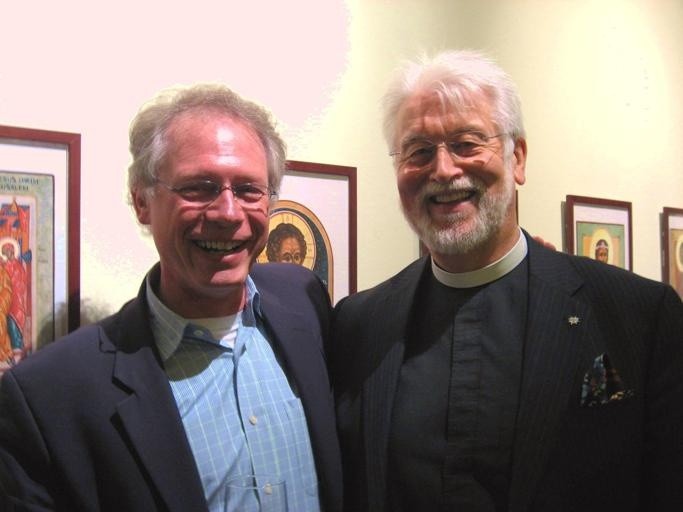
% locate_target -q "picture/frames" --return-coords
[0,124,81,381]
[563,195,683,304]
[253,160,357,318]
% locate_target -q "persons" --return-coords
[594,238,610,264]
[1,82,345,512]
[329,47,682,510]
[264,221,307,268]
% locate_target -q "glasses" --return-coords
[388,131,505,168]
[148,164,279,208]
